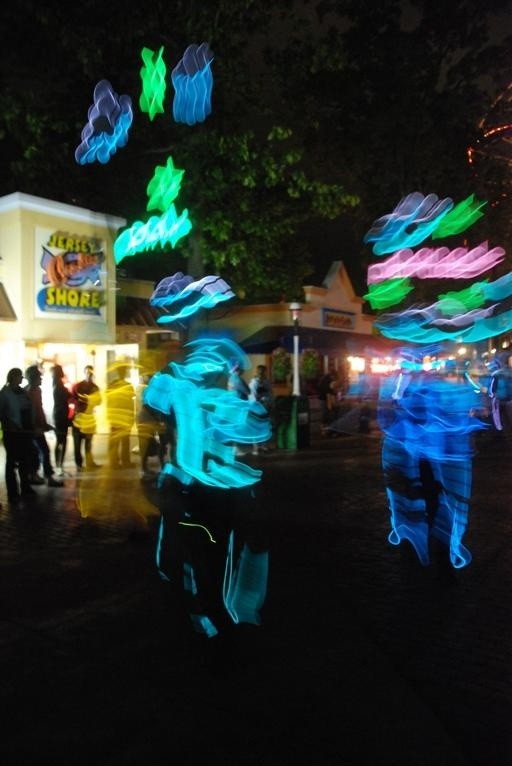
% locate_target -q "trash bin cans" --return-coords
[274,394,310,451]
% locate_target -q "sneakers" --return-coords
[48,479,64,487]
[28,477,45,484]
[20,490,35,505]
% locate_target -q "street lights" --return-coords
[287,301,304,399]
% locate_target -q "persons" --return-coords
[245,363,275,458]
[24,367,68,488]
[49,364,77,481]
[66,366,104,471]
[224,359,254,457]
[106,364,138,471]
[0,367,41,505]
[315,368,344,439]
